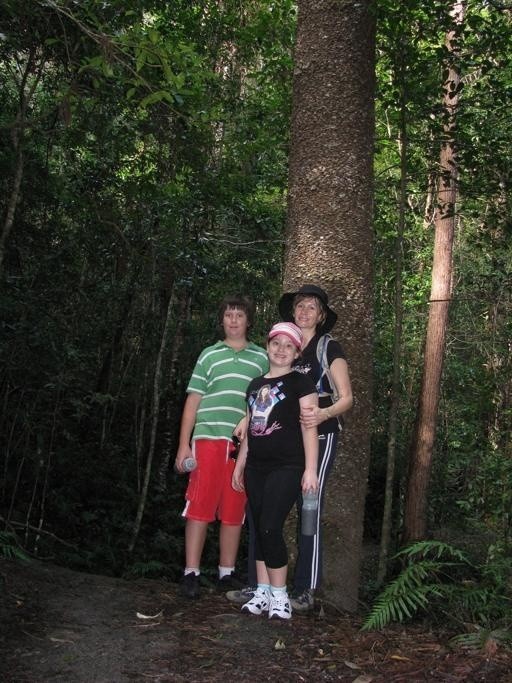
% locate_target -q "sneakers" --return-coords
[292,591,319,613]
[240,587,293,622]
[173,572,203,600]
[218,572,254,605]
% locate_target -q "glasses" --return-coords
[230,434,240,461]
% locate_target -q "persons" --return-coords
[174,295,271,600]
[232,321,320,621]
[226,284,354,615]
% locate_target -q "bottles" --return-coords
[301,485,319,536]
[173,457,197,475]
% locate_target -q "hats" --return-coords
[268,321,303,349]
[277,283,338,337]
